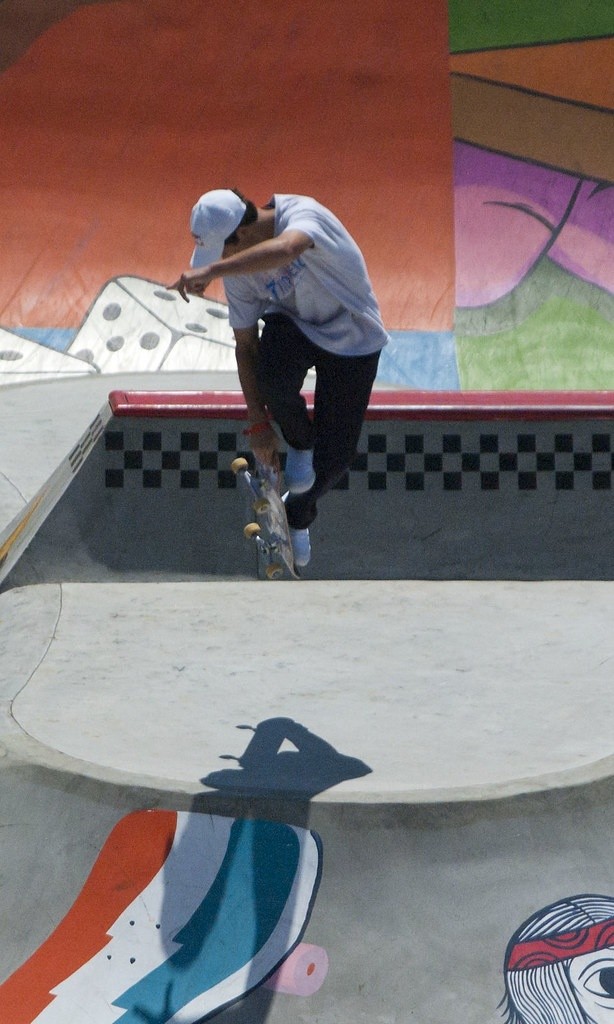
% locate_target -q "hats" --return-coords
[189,188,246,269]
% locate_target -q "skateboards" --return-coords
[228,451,303,583]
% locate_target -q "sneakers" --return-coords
[280,490,311,566]
[284,446,316,493]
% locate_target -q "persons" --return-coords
[166,189,392,568]
